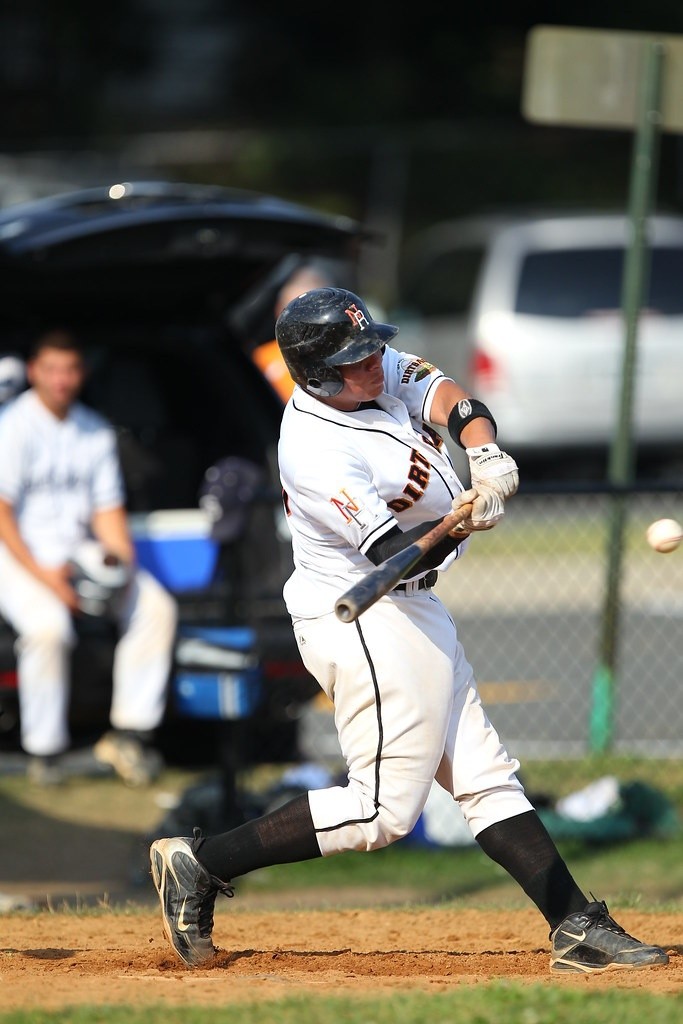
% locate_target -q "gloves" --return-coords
[465,443,520,503]
[443,486,505,535]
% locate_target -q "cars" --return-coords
[1,179,373,493]
[362,205,683,479]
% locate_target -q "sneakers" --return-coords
[548,890,670,972]
[151,826,236,971]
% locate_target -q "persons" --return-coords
[133,258,370,857]
[148,289,670,978]
[0,328,179,791]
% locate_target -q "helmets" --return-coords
[276,287,399,398]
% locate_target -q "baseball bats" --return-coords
[333,500,473,624]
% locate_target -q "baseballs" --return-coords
[646,518,683,553]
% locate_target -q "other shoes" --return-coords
[94,730,162,786]
[27,752,67,787]
